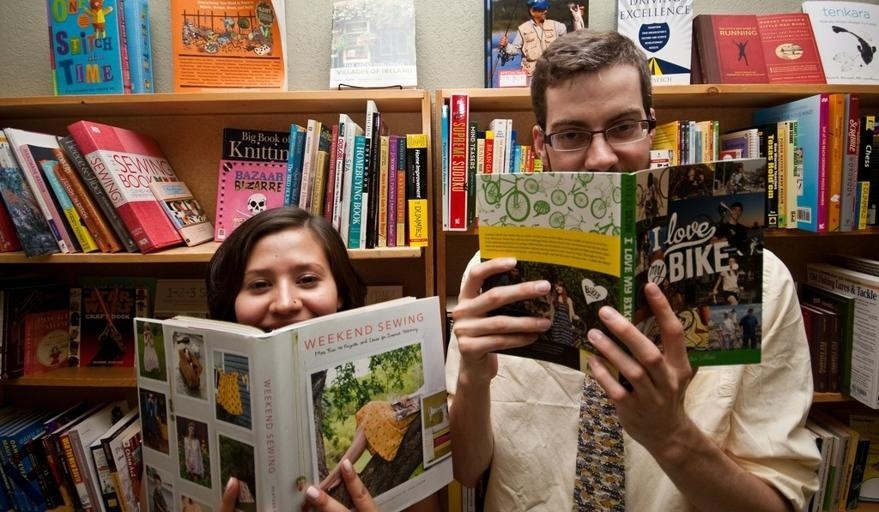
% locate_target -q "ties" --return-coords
[571,374,626,512]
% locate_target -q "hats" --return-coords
[730,201,745,209]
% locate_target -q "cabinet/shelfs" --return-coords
[436,85,875,512]
[0,89,433,512]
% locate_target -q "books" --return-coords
[67,278,155,368]
[46,1,155,96]
[282,101,429,249]
[134,296,452,512]
[800,2,879,86]
[331,3,418,89]
[214,128,292,245]
[172,2,287,93]
[0,400,143,512]
[767,94,878,233]
[442,98,476,233]
[758,15,827,85]
[0,278,69,380]
[796,257,879,410]
[806,414,879,511]
[484,1,588,87]
[694,15,767,84]
[0,122,214,258]
[479,118,544,173]
[650,122,768,160]
[480,159,765,389]
[158,279,207,321]
[618,1,693,85]
[362,285,404,304]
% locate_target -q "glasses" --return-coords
[542,115,659,153]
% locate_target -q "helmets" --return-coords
[526,0,550,11]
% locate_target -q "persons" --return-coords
[545,279,581,346]
[725,164,752,194]
[718,312,736,351]
[177,337,201,388]
[713,259,740,306]
[222,453,256,512]
[318,385,423,490]
[718,205,751,238]
[501,0,583,86]
[153,474,168,512]
[143,321,161,373]
[143,392,161,446]
[739,309,760,351]
[184,420,204,480]
